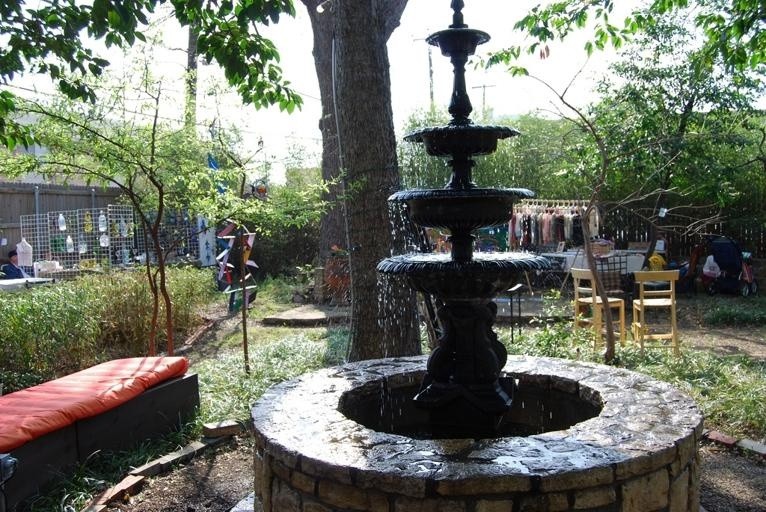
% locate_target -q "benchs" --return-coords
[0,356,199,511]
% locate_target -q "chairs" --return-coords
[569,267,681,357]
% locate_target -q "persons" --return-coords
[2,250,31,278]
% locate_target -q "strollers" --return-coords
[695,230,758,299]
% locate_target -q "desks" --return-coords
[541,249,666,290]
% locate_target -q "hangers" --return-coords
[518,199,594,214]
